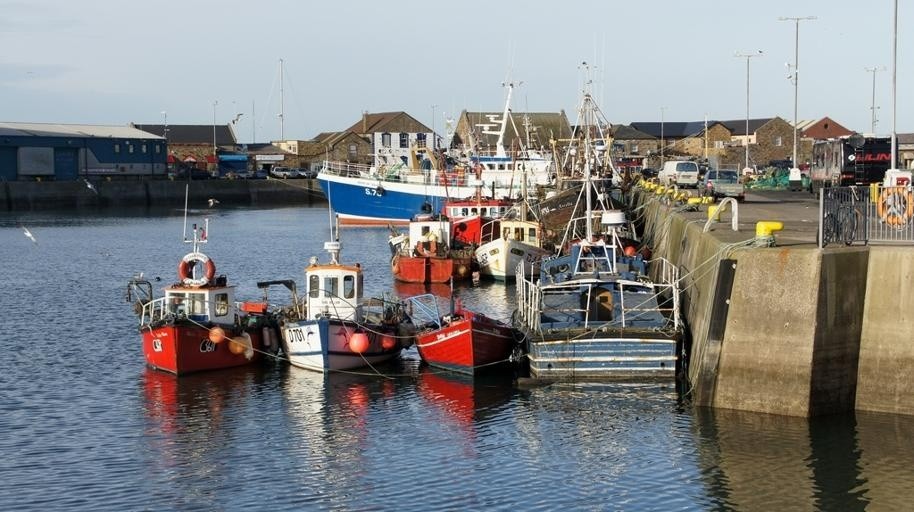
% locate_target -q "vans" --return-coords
[658,161,699,187]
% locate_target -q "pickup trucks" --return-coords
[697,168,746,204]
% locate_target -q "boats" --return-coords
[125,187,275,377]
[314,81,560,227]
[277,142,399,374]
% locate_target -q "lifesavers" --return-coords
[877,186,914,225]
[178,252,215,288]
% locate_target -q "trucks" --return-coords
[809,136,899,196]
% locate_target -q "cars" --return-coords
[179,165,209,179]
[256,170,267,179]
[272,164,311,180]
[641,168,658,179]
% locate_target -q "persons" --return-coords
[474,164,482,180]
[581,246,594,270]
[417,241,438,257]
[515,231,520,240]
[504,228,509,240]
[386,303,400,323]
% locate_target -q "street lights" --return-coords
[736,50,766,167]
[779,15,817,167]
[864,64,883,135]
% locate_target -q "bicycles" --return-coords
[816,192,859,247]
